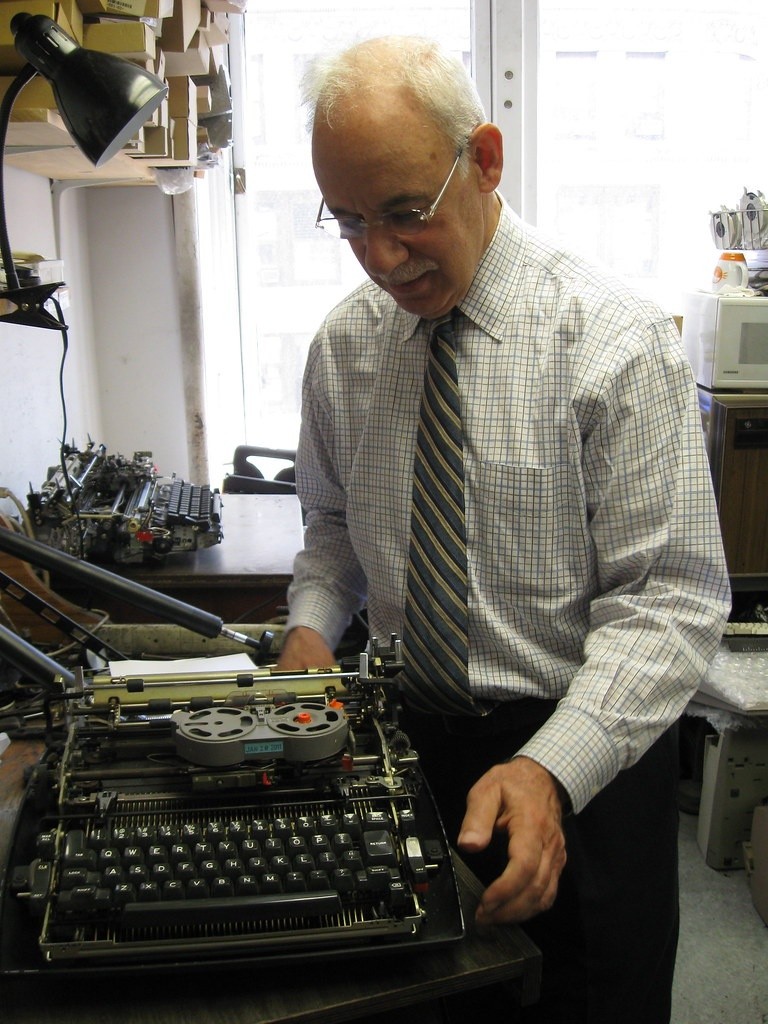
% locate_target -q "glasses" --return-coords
[315,150,462,238]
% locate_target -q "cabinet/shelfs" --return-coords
[38,493,304,625]
[696,388,768,594]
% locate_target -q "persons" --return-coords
[277,38,731,1024]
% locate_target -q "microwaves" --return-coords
[681,291,768,392]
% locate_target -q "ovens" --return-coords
[696,386,768,592]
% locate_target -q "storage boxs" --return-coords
[0,0,245,168]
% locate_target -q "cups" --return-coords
[713,253,748,295]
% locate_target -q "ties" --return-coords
[399,316,482,721]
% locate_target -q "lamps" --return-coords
[0,11,169,330]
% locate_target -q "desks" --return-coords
[0,732,543,1023]
